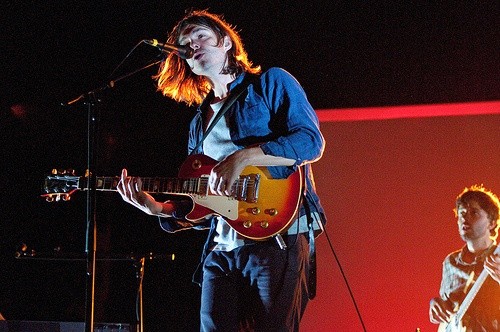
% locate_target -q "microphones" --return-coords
[143,39,195,59]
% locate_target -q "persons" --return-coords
[115,9,326,332]
[427,185,500,332]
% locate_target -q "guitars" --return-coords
[436,240,500,332]
[43,151,306,241]
